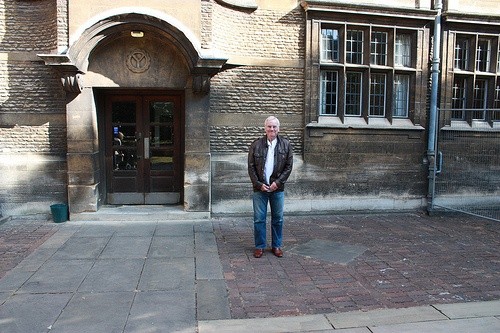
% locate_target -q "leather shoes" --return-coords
[272,248,283,257]
[254,248,263,257]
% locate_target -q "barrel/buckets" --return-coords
[50,204,68,223]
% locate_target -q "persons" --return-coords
[112,126,160,172]
[247,115,293,258]
